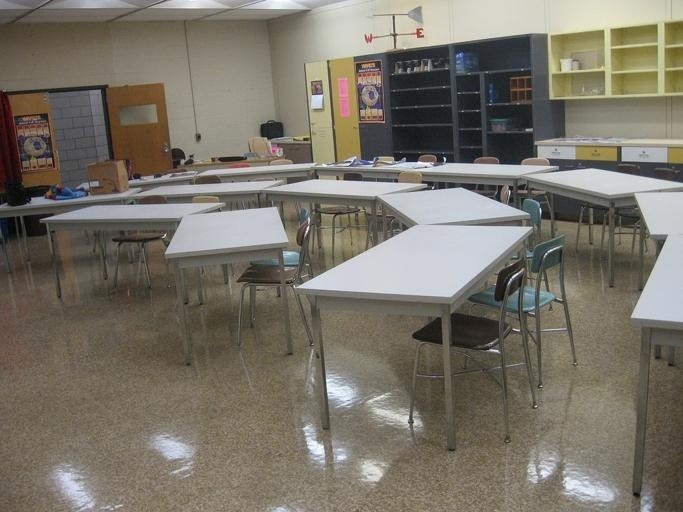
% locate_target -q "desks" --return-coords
[271,140,313,164]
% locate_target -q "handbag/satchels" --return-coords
[259,119,285,140]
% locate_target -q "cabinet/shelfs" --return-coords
[547,19,682,101]
[451,33,565,165]
[384,42,454,161]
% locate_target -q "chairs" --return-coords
[417,155,438,163]
[374,157,394,162]
[172,148,185,168]
